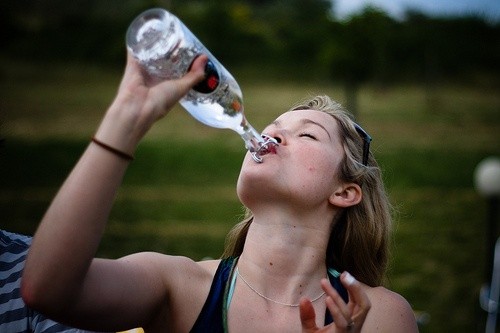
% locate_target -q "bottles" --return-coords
[124,9,279,162]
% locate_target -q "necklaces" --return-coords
[236,268,326,307]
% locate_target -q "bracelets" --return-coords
[89,135,134,160]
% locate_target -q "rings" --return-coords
[348,320,353,331]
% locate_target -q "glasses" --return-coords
[351,121,372,182]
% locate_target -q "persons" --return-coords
[0,230,93,333]
[20,47,420,333]
